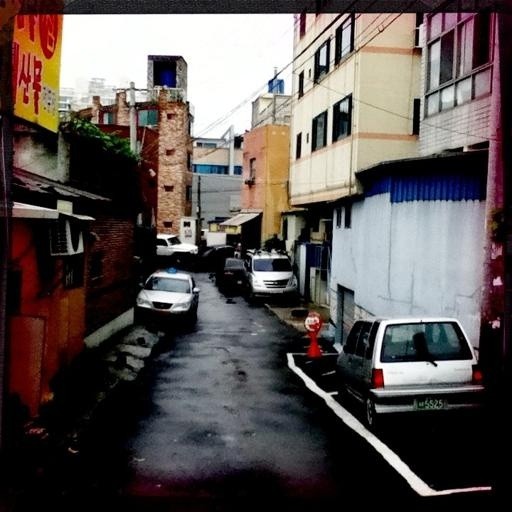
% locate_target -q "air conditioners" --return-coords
[49,219,85,256]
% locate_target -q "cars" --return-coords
[157,234,200,269]
[135,267,200,329]
[336,317,487,432]
[200,234,299,301]
